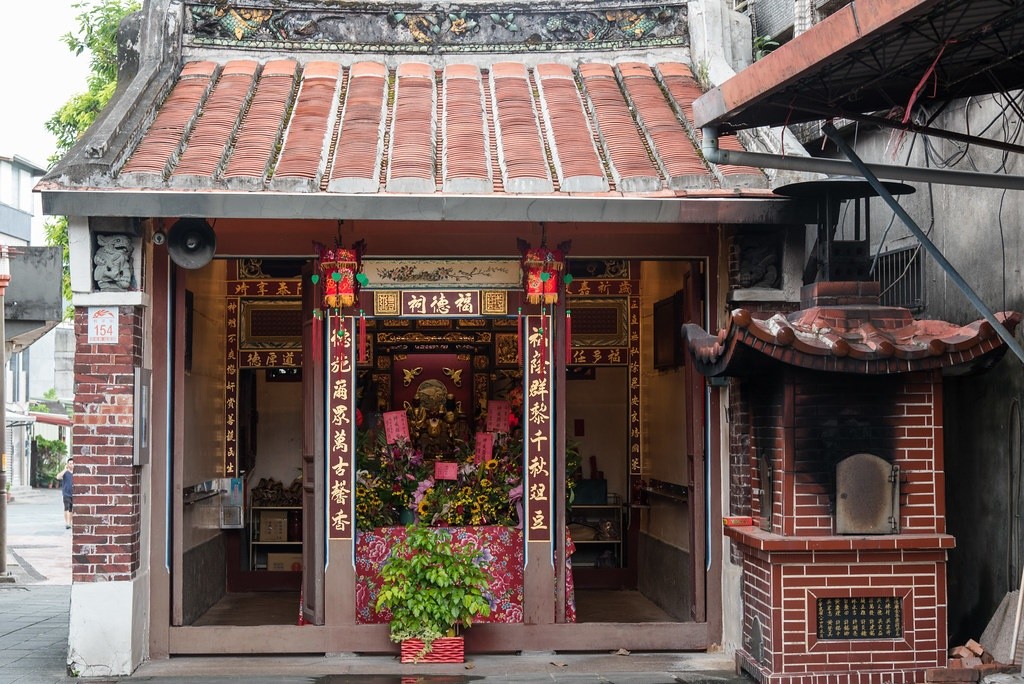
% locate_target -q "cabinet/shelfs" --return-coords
[250,494,303,572]
[565,504,623,568]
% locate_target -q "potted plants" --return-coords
[373,523,496,662]
[34,468,59,488]
[6,481,12,502]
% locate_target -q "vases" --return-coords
[399,509,415,526]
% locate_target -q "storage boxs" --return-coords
[257,510,288,542]
[268,553,303,572]
[723,516,752,526]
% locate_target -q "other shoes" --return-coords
[65,523,70,529]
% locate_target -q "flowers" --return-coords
[355,385,524,532]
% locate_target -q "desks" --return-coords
[298,525,575,624]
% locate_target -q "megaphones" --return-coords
[166,217,217,269]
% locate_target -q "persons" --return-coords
[56,458,74,529]
